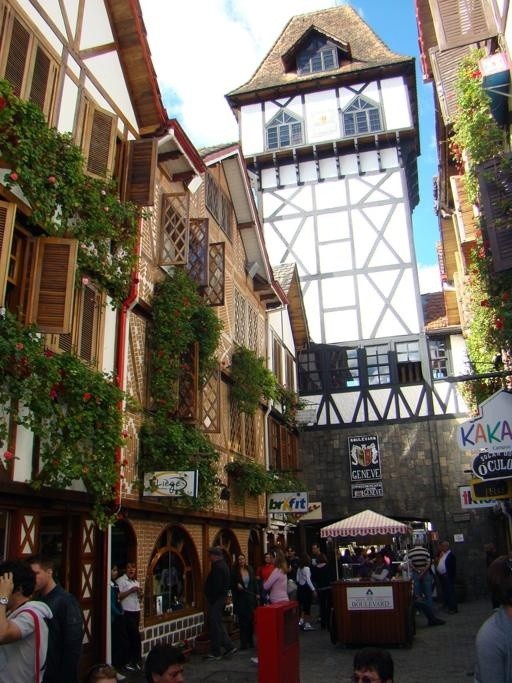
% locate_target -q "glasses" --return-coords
[350,674,390,683]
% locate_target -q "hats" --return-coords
[207,547,224,556]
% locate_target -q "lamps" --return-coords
[210,473,230,501]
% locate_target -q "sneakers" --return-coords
[427,616,447,627]
[222,647,239,660]
[202,653,222,663]
[297,618,321,632]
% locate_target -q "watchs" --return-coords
[1,595,9,606]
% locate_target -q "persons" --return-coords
[0,557,53,683]
[116,561,145,671]
[472,555,511,683]
[111,563,130,681]
[351,646,393,683]
[26,553,83,682]
[146,643,186,683]
[201,539,460,660]
[84,663,117,683]
